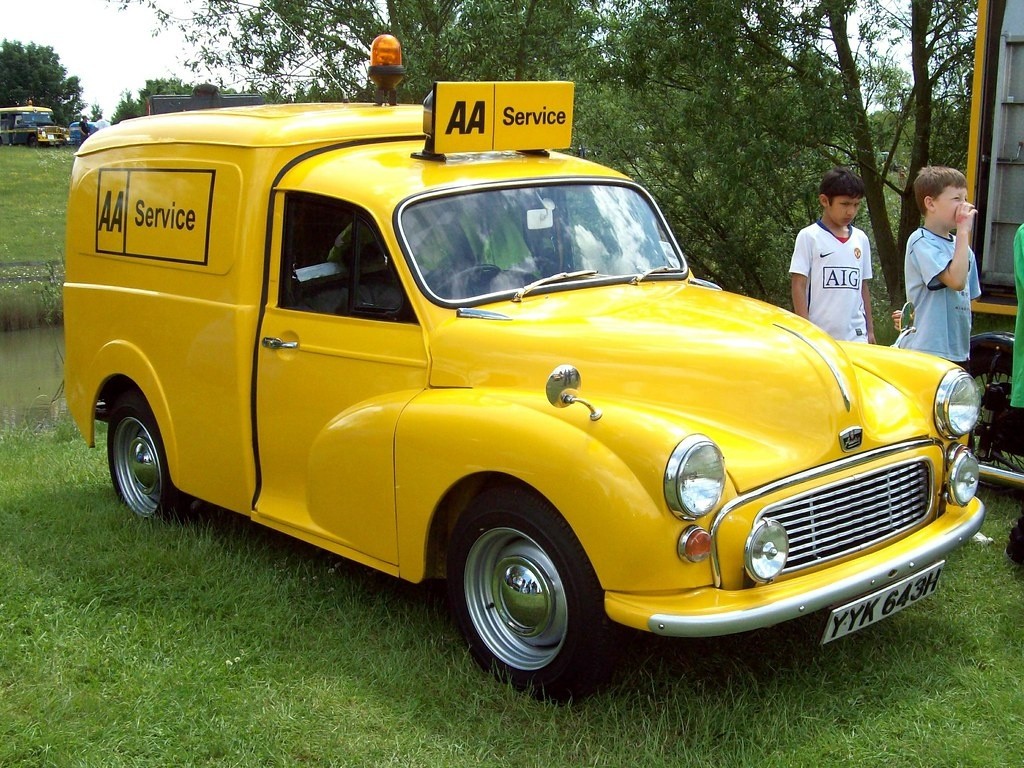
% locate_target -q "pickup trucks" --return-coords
[57,28,989,686]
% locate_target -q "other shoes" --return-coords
[971,531,990,545]
[1002,536,1023,562]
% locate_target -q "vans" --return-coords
[0,100,67,149]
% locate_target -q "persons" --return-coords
[78,116,89,142]
[93,114,107,132]
[1000,222,1024,568]
[787,166,878,345]
[890,165,995,550]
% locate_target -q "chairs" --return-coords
[327,217,422,316]
